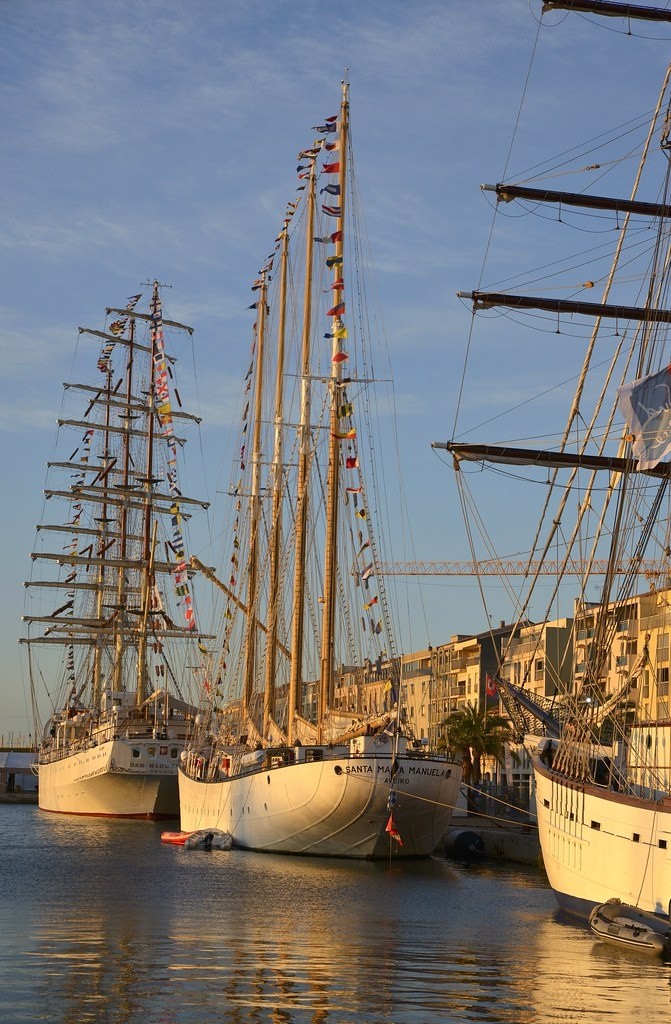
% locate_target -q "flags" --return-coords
[617,363,671,471]
[67,166,405,849]
[486,674,498,696]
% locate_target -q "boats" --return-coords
[588,897,671,956]
[161,828,232,851]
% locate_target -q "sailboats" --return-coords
[424,0,671,928]
[166,64,464,859]
[11,280,221,821]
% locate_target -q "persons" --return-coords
[50,726,56,737]
